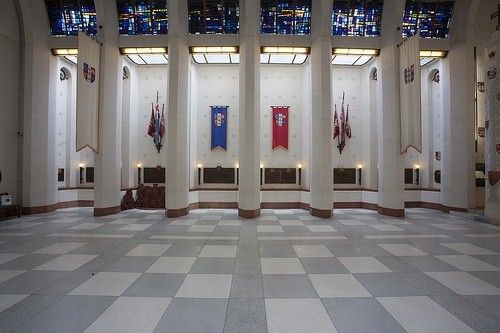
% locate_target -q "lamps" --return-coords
[358,165,362,185]
[79,163,84,184]
[137,164,142,184]
[298,164,302,185]
[198,164,202,185]
[260,165,263,185]
[416,165,420,185]
[236,163,239,185]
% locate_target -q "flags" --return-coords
[211,106,227,152]
[345,107,351,140]
[76,29,100,154]
[337,101,345,154]
[148,107,154,137]
[272,107,288,151]
[159,108,165,138]
[333,111,340,139]
[153,97,160,150]
[398,30,422,154]
